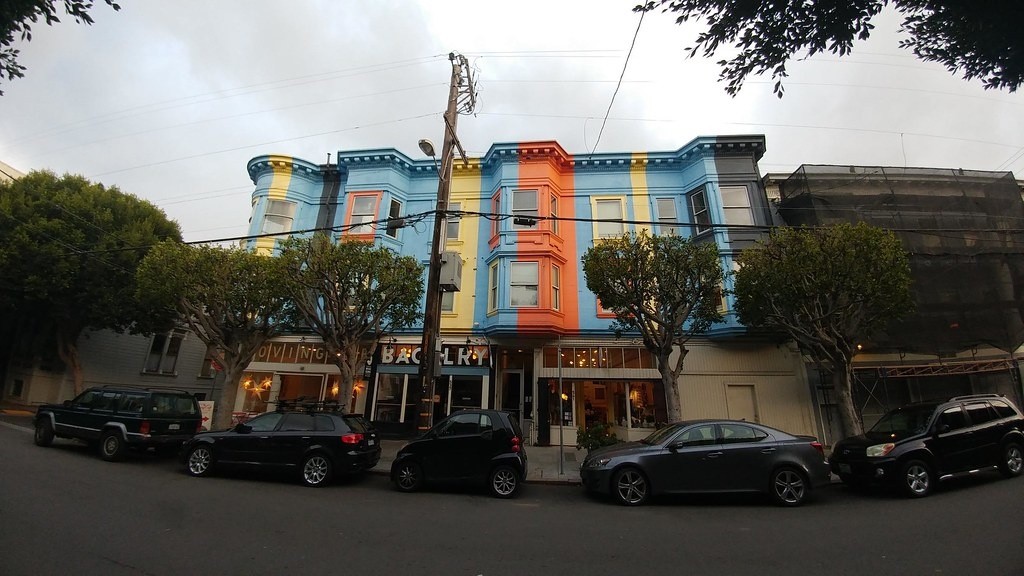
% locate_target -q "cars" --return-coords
[391,409,527,498]
[582,419,831,505]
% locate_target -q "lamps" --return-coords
[463,336,471,355]
[386,336,397,350]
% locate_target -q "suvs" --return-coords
[32,384,203,461]
[179,397,382,486]
[828,394,1024,497]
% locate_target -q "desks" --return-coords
[231,412,246,427]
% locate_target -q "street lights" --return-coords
[420,137,448,435]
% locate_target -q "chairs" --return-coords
[247,411,259,419]
[686,429,703,440]
